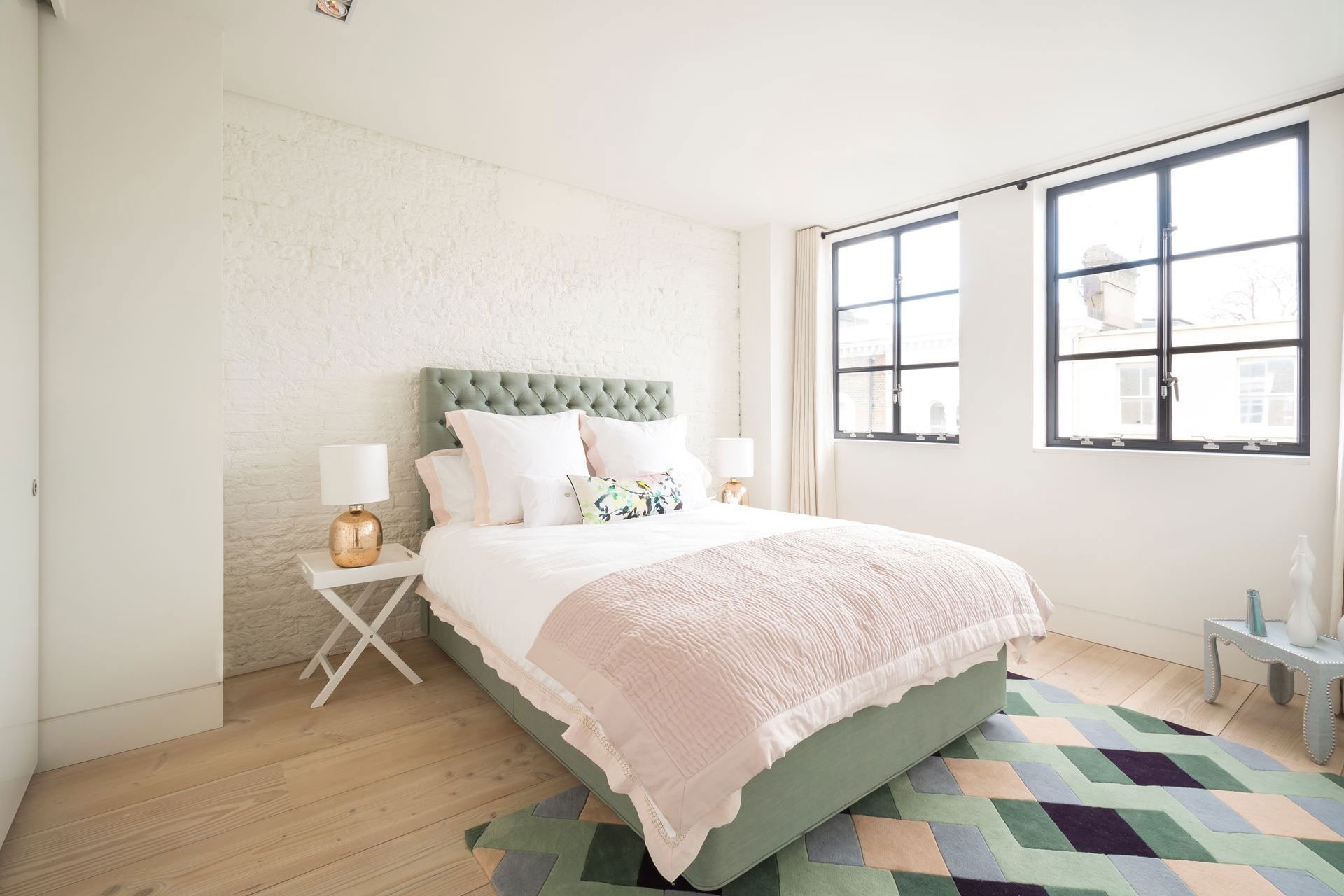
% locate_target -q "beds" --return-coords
[415,367,1055,891]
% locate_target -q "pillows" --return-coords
[414,409,713,529]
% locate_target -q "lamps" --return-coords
[716,438,754,506]
[318,444,390,568]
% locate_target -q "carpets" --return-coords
[465,669,1344,896]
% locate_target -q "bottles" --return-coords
[1246,588,1266,637]
[1291,534,1323,641]
[1286,552,1318,649]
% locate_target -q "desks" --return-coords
[297,543,426,709]
[1203,618,1344,765]
